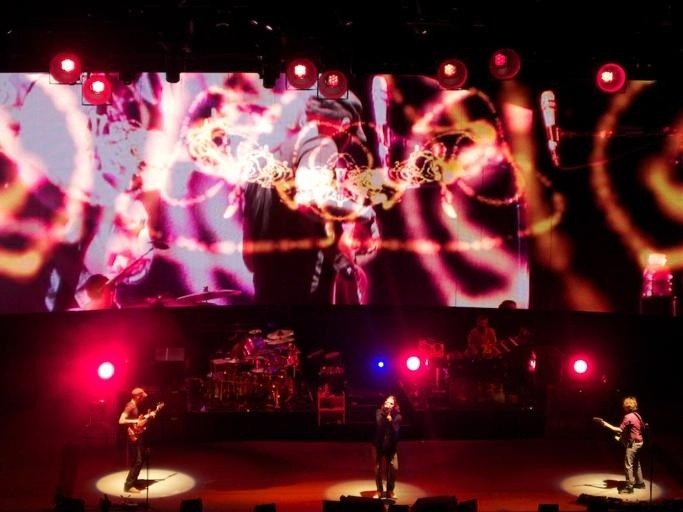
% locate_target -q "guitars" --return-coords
[127,402,165,443]
[592,417,629,447]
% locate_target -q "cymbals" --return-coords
[266,329,294,339]
[264,338,295,345]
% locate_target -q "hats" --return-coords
[131,388,148,398]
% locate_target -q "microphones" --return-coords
[372,76,391,167]
[537,90,561,168]
[151,241,169,249]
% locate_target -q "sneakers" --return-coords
[124,486,140,494]
[373,488,398,500]
[622,483,647,492]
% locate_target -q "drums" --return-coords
[241,355,265,375]
[211,358,241,385]
[269,352,289,378]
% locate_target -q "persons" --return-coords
[466,314,496,403]
[369,395,402,499]
[118,388,158,492]
[594,396,649,494]
